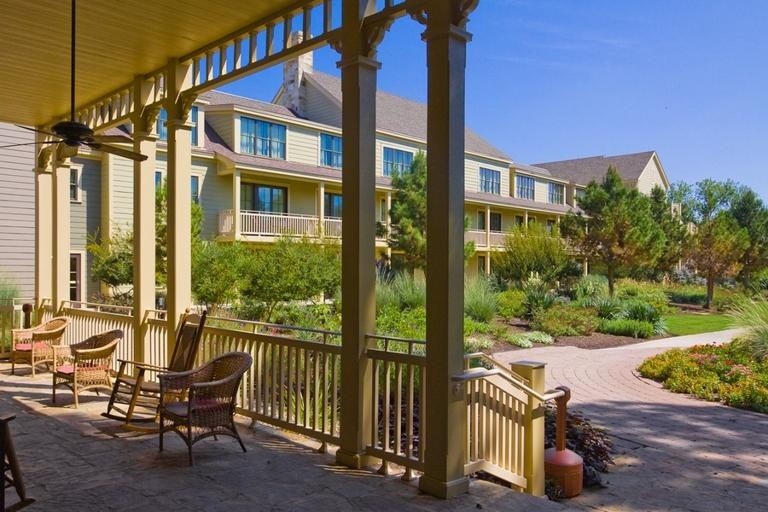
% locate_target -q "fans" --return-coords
[1,0,149,160]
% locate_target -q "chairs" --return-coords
[101,308,207,432]
[51,329,123,408]
[10,315,71,377]
[0,412,36,512]
[156,351,252,467]
